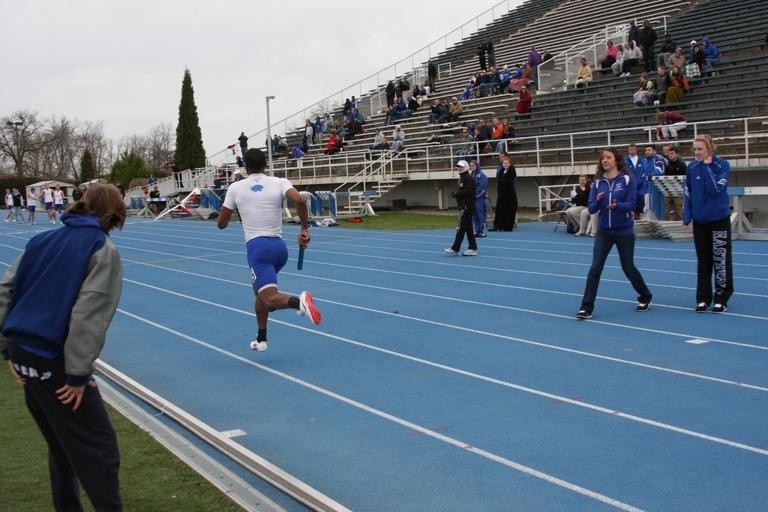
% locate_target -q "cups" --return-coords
[654,101,659,105]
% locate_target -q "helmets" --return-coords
[456,160,469,173]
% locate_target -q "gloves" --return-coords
[448,189,457,198]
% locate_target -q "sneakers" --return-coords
[712,304,727,313]
[637,296,652,313]
[575,233,585,237]
[462,250,477,256]
[576,310,592,319]
[589,233,596,236]
[696,304,708,312]
[297,292,321,325]
[250,340,266,351]
[442,248,458,256]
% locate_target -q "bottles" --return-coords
[656,135,660,140]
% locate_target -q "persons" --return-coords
[1,182,126,512]
[574,146,654,319]
[238,96,366,167]
[217,148,322,351]
[680,133,734,314]
[623,143,687,219]
[600,21,720,143]
[366,39,554,160]
[565,176,601,237]
[443,150,518,257]
[163,160,185,188]
[0,180,162,227]
[575,58,593,94]
